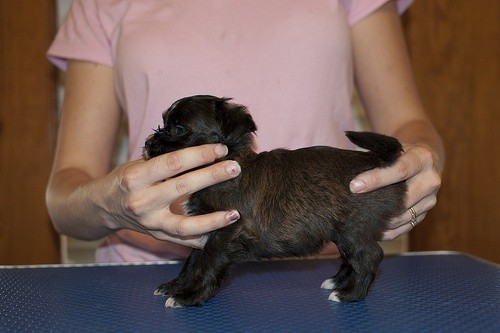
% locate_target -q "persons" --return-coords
[46,0,446,264]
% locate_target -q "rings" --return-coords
[409,207,416,221]
[411,219,419,227]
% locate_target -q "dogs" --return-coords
[144,94,408,309]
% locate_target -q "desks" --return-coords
[0,249,500,333]
